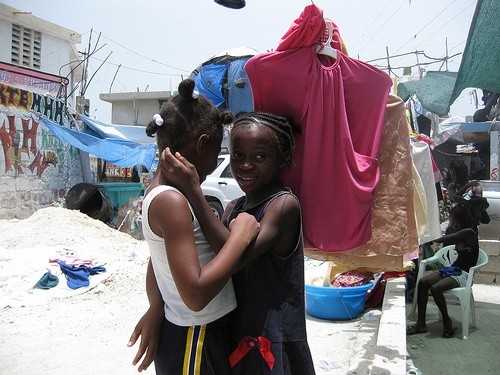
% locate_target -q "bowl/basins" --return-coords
[304,281,374,320]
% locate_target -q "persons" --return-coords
[406,204,478,339]
[441,163,483,245]
[129,77,315,375]
[439,167,451,189]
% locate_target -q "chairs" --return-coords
[408,246,488,340]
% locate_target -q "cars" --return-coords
[200,154,246,221]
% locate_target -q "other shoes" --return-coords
[407,325,427,333]
[442,329,456,338]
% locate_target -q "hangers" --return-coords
[316,17,337,59]
